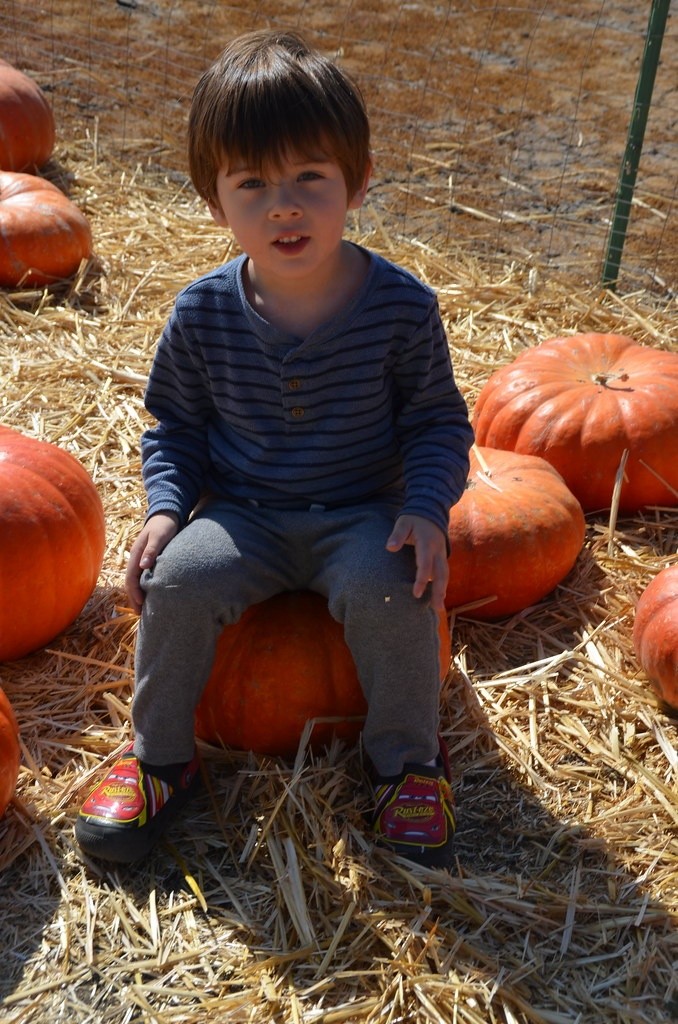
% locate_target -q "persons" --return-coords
[77,27,477,866]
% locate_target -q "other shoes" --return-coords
[357,722,458,868]
[75,740,207,863]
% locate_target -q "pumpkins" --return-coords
[0,685,21,824]
[0,57,56,175]
[0,169,93,289]
[444,447,586,620]
[633,564,678,712]
[469,332,678,513]
[192,589,452,753]
[0,424,107,662]
